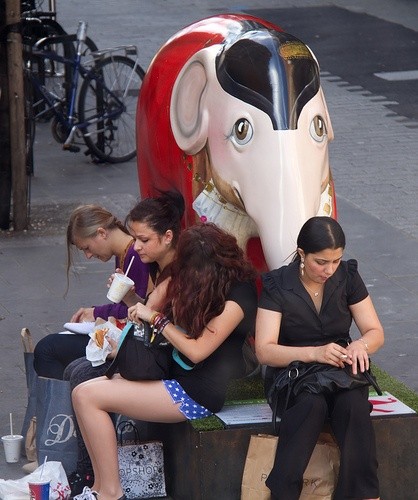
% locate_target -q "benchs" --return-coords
[148,361,418,500]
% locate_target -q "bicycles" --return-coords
[0,0,147,175]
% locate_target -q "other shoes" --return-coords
[71,485,127,500]
[21,461,38,473]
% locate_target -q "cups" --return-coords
[106,273,135,304]
[1,434,24,462]
[27,479,50,500]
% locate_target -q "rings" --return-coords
[339,354,347,360]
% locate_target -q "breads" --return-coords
[94,327,110,347]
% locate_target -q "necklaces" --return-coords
[301,279,323,296]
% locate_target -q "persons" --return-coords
[70,223,258,500]
[63,189,185,496]
[255,216,384,500]
[21,204,152,473]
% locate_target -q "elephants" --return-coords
[134,12,340,349]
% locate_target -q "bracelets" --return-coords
[359,338,368,351]
[150,313,170,334]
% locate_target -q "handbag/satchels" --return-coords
[269,337,382,436]
[0,327,167,500]
[104,322,195,382]
